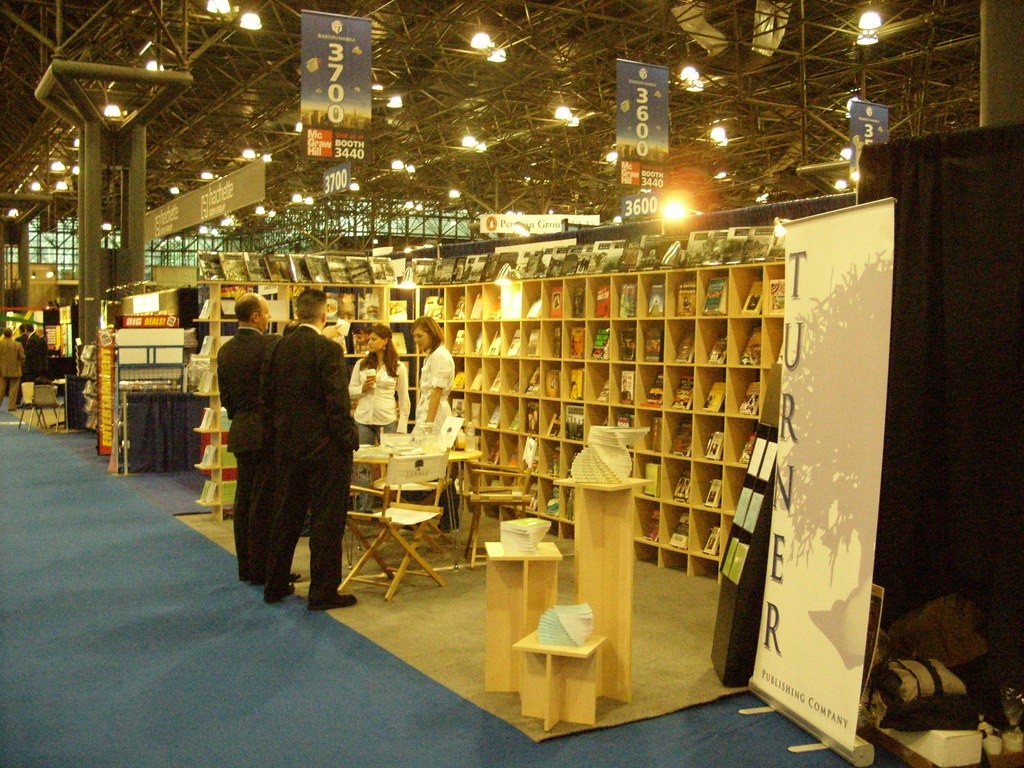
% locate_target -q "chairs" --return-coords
[27,385,66,433]
[458,436,536,570]
[19,382,48,430]
[338,426,451,601]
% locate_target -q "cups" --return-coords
[335,318,350,336]
[365,368,377,388]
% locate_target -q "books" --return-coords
[197,298,216,507]
[219,235,783,556]
[198,250,226,281]
[79,345,98,432]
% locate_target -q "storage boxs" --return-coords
[875,722,984,767]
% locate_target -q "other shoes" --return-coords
[291,573,301,581]
[307,592,357,611]
[264,583,295,603]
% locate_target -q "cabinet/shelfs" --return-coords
[80,262,786,588]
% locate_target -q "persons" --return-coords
[282,319,301,335]
[348,324,412,514]
[263,290,357,613]
[0,323,42,413]
[411,316,459,532]
[217,293,303,584]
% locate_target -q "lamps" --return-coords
[31,271,37,279]
[661,240,685,268]
[513,222,531,237]
[401,267,417,289]
[494,263,516,286]
[773,218,788,238]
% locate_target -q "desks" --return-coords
[346,435,482,569]
[553,478,653,702]
[484,542,563,695]
[512,631,609,734]
[52,380,68,431]
